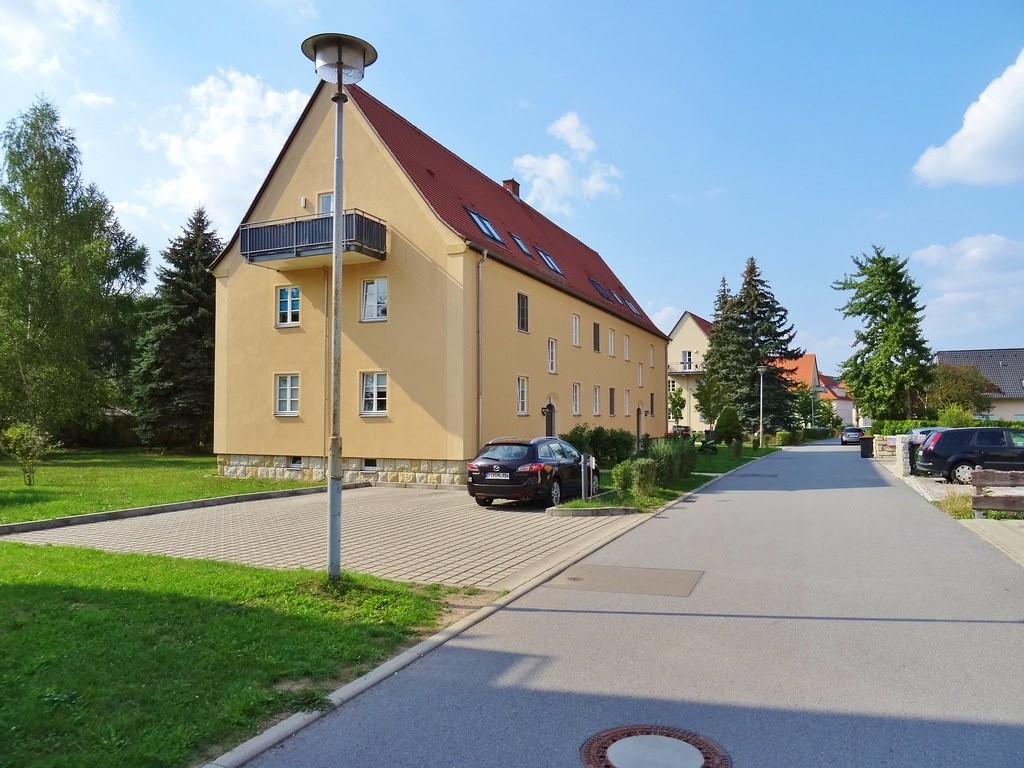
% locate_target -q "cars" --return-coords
[669,425,696,441]
[861,425,872,434]
[840,427,865,445]
[904,426,947,445]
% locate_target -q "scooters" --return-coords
[690,434,718,454]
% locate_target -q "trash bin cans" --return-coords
[858,435,875,457]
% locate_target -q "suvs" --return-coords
[464,434,601,508]
[915,424,1024,485]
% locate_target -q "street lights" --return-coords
[755,365,768,448]
[808,395,814,428]
[299,31,380,583]
